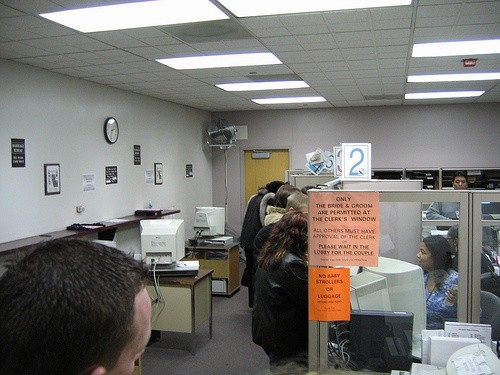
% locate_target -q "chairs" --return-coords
[481,271,500,296]
[480,289,500,340]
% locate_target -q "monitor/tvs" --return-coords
[350,256,426,341]
[140,219,185,265]
[193,207,225,236]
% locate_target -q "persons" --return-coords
[416,235,482,331]
[446,224,495,273]
[0,239,153,375]
[242,181,316,375]
[426,172,472,220]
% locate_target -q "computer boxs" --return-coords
[196,236,233,246]
[348,310,414,371]
[146,260,200,277]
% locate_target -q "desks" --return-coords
[138,269,215,355]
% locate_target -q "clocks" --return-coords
[104,116,120,144]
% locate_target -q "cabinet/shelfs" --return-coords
[182,241,241,298]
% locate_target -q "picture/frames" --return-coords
[154,162,164,185]
[43,162,62,196]
